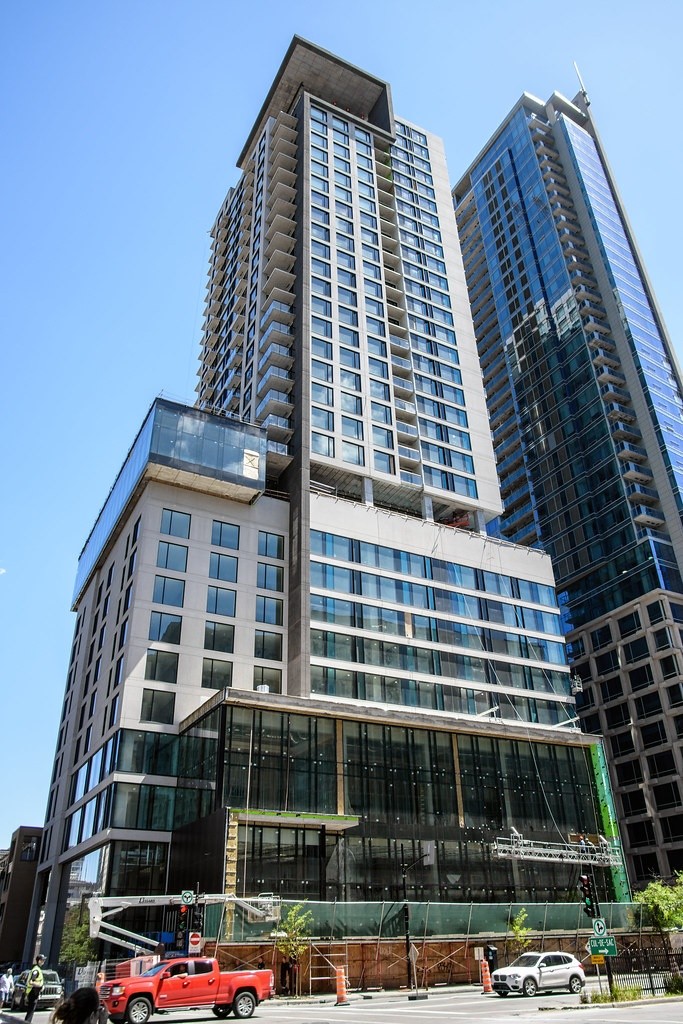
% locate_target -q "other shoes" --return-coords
[288,990,290,996]
[280,993,284,997]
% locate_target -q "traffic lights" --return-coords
[175,903,189,951]
[578,873,598,918]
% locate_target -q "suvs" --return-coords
[490,951,586,998]
[11,969,66,1011]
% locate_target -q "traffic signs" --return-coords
[589,936,618,956]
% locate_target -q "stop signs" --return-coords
[190,933,201,945]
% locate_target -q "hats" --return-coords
[36,954,47,960]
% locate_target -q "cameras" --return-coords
[97,1006,105,1020]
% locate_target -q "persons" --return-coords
[171,965,188,979]
[577,836,596,860]
[235,959,243,971]
[53,987,109,1024]
[0,968,15,1009]
[258,956,265,970]
[24,954,47,1024]
[280,956,300,997]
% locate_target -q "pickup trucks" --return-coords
[98,955,277,1024]
